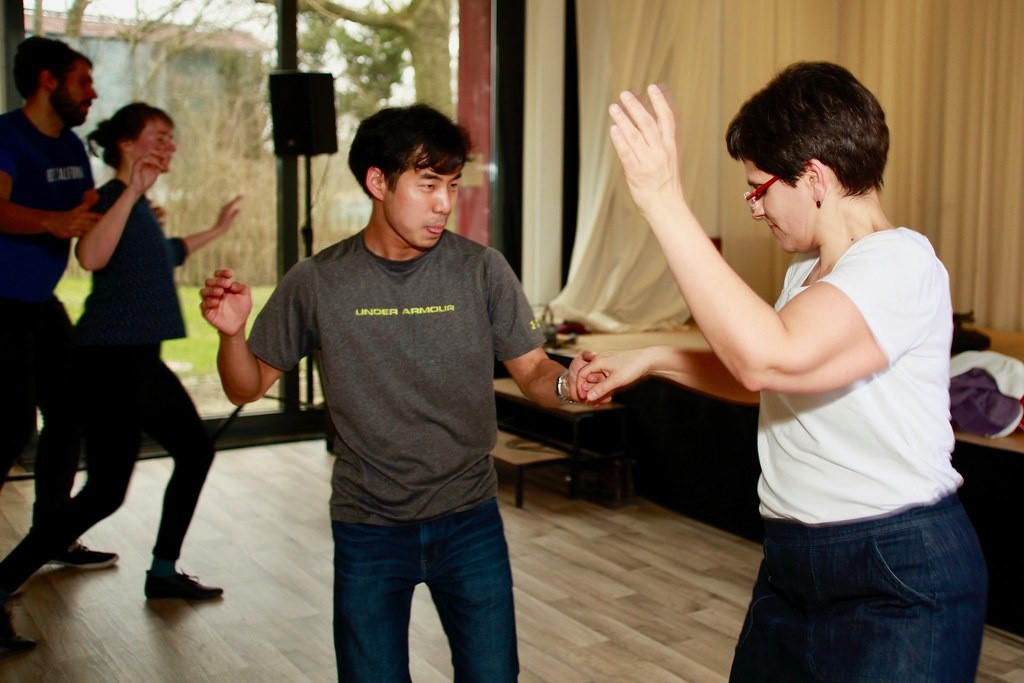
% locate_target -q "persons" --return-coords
[199,102,613,683]
[575,60,988,682]
[0,35,120,569]
[0,102,244,657]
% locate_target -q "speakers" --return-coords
[268,70,339,158]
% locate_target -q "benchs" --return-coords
[489,429,573,509]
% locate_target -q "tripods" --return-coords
[212,156,317,443]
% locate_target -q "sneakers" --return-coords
[46,538,119,568]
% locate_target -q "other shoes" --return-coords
[145,568,223,599]
[0,609,37,650]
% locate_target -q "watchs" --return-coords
[555,370,574,405]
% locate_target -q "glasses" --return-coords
[743,176,779,210]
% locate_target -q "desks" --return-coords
[493,379,624,500]
